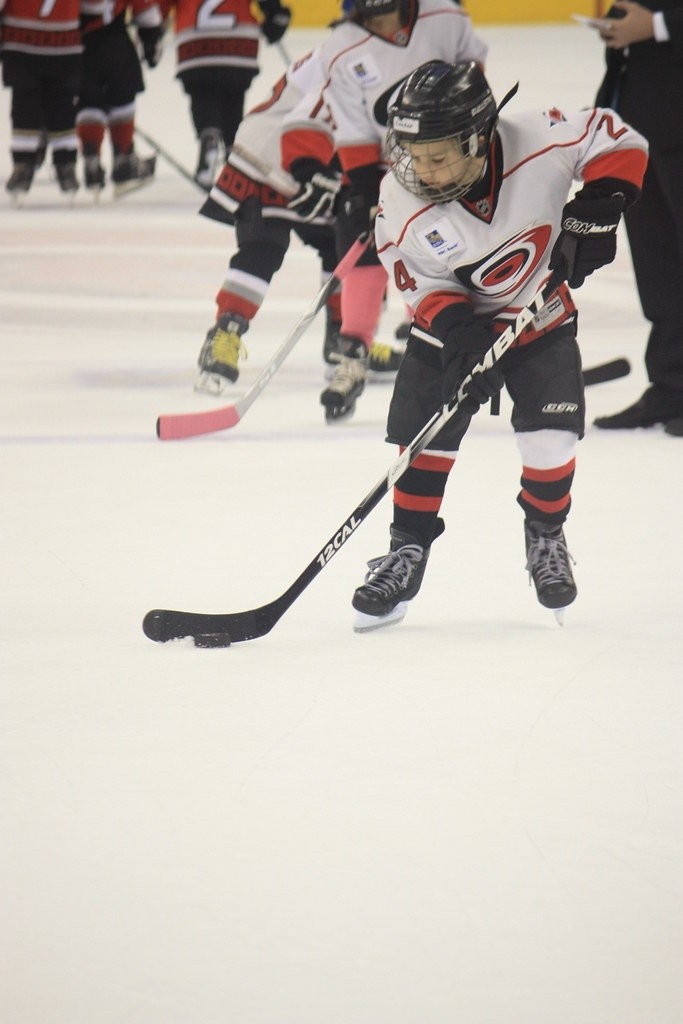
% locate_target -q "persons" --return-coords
[593,1,683,437]
[0,0,170,209]
[351,57,649,635]
[176,0,291,185]
[279,0,490,425]
[191,0,403,397]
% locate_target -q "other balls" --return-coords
[193,632,232,647]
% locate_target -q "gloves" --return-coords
[261,7,291,44]
[441,346,504,415]
[332,191,377,227]
[286,183,334,221]
[138,26,162,59]
[548,193,623,289]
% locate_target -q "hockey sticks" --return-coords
[140,268,566,643]
[132,125,209,195]
[155,225,376,439]
[275,39,294,69]
[581,358,631,385]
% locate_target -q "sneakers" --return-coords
[6,164,36,204]
[352,517,446,634]
[198,313,249,395]
[524,518,578,627]
[323,321,403,382]
[83,143,106,201]
[56,163,81,202]
[320,334,368,424]
[195,132,219,190]
[111,146,158,197]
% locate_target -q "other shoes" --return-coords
[594,382,683,429]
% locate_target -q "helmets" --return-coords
[385,60,498,205]
